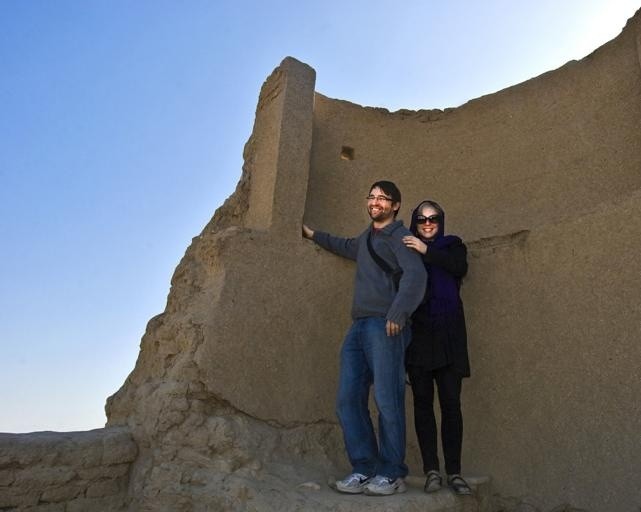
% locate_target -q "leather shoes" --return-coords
[423,472,444,494]
[447,472,472,497]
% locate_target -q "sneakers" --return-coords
[335,468,374,494]
[364,474,408,496]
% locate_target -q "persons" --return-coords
[403,200,474,495]
[303,179,429,497]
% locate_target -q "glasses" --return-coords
[414,212,442,226]
[366,191,397,204]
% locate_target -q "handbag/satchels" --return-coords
[392,271,429,307]
